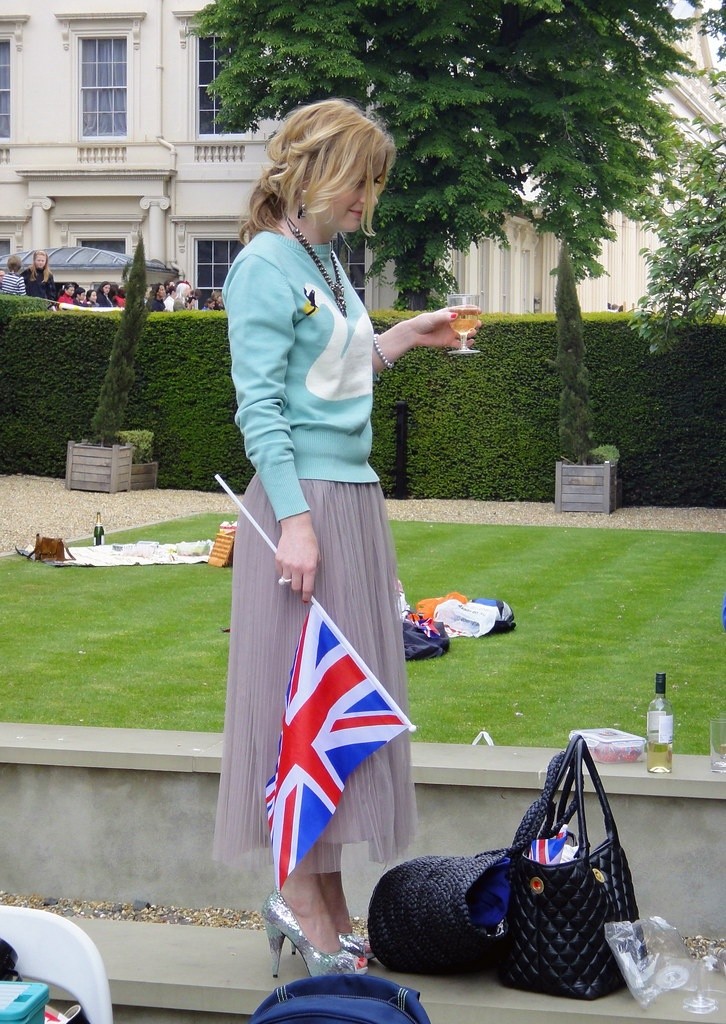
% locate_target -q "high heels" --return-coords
[261,891,377,975]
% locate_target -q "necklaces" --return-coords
[284,213,348,318]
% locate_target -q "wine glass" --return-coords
[447,294,481,354]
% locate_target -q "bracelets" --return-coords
[373,333,395,369]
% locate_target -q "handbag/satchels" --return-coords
[401,592,512,661]
[498,730,646,999]
[367,746,584,973]
[40,538,74,560]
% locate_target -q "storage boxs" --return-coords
[0,980,50,1024]
[569,728,647,763]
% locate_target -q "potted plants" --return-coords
[64,229,148,493]
[554,236,620,515]
[116,430,158,490]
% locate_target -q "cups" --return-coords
[710,719,726,773]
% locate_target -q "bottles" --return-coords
[645,673,672,774]
[93,512,104,546]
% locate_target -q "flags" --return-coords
[264,599,407,895]
[527,834,567,864]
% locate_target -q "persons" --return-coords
[58,282,75,304]
[20,250,55,301]
[97,281,116,307]
[74,287,88,307]
[208,99,480,979]
[2,256,27,296]
[0,269,6,291]
[83,290,100,307]
[111,279,225,312]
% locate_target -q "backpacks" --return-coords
[247,977,429,1024]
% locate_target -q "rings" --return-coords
[277,576,291,587]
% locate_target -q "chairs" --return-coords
[0,904,114,1024]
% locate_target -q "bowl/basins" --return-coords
[569,727,646,764]
